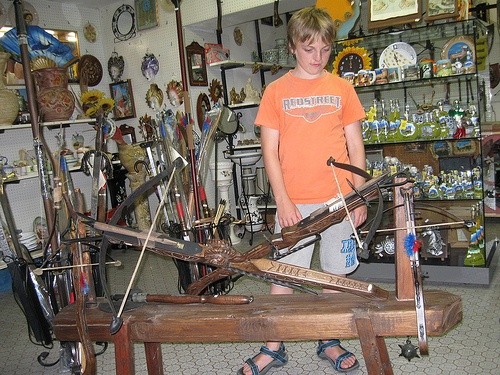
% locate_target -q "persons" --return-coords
[238,7,367,375]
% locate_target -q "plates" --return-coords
[378,40,418,79]
[330,44,373,78]
[18,232,39,250]
[440,34,476,73]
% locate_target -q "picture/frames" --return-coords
[186,41,208,85]
[110,79,136,121]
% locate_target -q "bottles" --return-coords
[245,196,263,232]
[365,156,483,201]
[274,38,289,65]
[463,201,486,266]
[0,52,20,126]
[32,65,76,123]
[359,96,481,144]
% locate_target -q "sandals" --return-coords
[237,342,288,375]
[316,339,359,372]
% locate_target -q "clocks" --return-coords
[440,34,476,74]
[331,46,372,78]
[378,42,417,70]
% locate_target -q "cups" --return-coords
[344,58,477,88]
[261,49,279,64]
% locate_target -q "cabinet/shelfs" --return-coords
[1,119,126,296]
[325,17,497,287]
